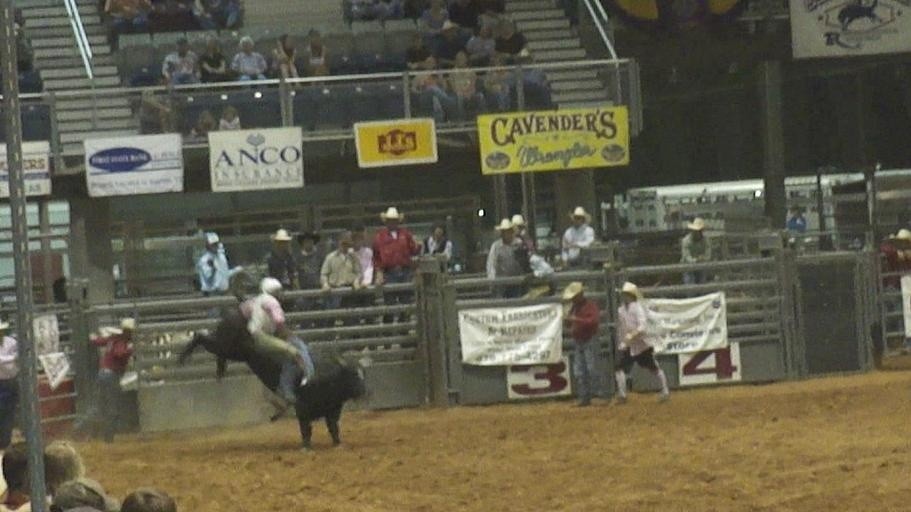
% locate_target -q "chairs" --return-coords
[0,1,562,138]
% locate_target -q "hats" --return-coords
[272,229,319,244]
[494,215,527,231]
[687,218,711,230]
[259,278,282,293]
[205,233,218,245]
[568,207,591,223]
[895,229,910,240]
[615,282,638,296]
[563,282,588,300]
[42,442,120,512]
[380,207,403,224]
[119,317,134,329]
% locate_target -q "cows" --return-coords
[180,271,371,451]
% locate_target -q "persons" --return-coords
[269,207,419,353]
[1,447,48,511]
[304,29,329,87]
[49,477,121,511]
[184,227,242,319]
[270,34,300,87]
[218,105,240,131]
[559,282,612,405]
[138,88,182,135]
[40,440,83,496]
[486,215,555,299]
[879,227,910,358]
[121,489,176,511]
[127,36,231,94]
[562,205,594,270]
[336,32,362,74]
[232,35,268,89]
[1,316,27,452]
[849,234,865,248]
[611,279,672,404]
[672,213,713,287]
[242,277,315,416]
[71,314,135,442]
[790,204,808,237]
[97,1,242,54]
[189,109,218,136]
[343,1,530,123]
[419,224,451,263]
